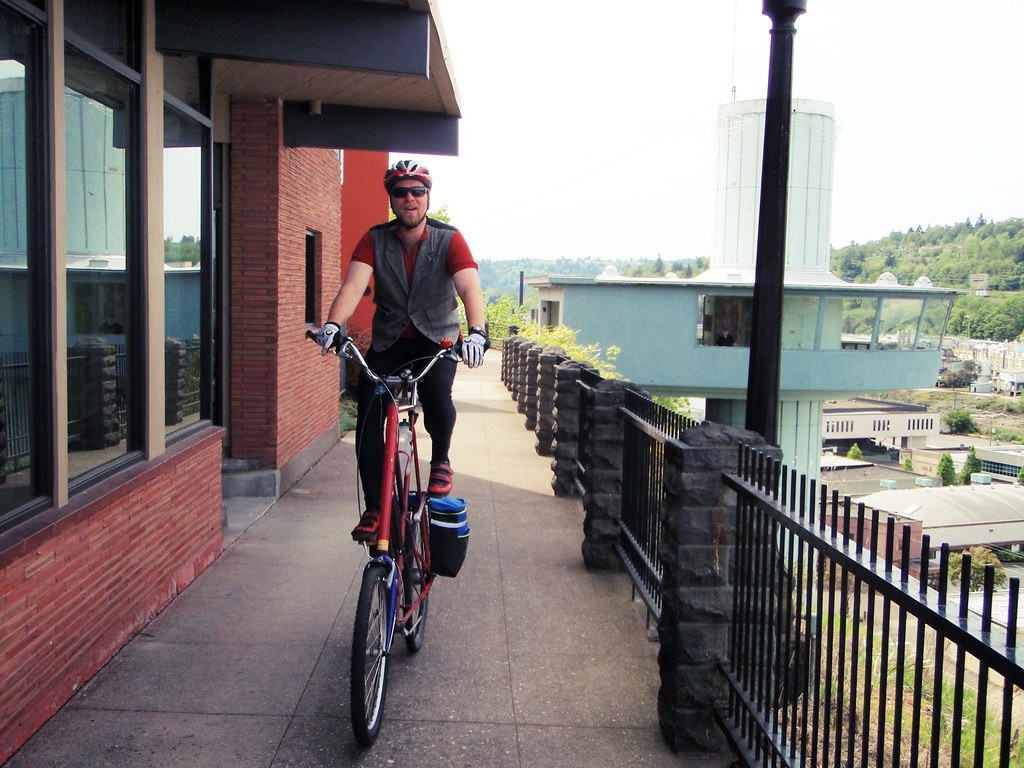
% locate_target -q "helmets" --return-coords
[383,160,432,195]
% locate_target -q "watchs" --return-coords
[468,325,486,338]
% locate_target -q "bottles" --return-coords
[398,418,412,474]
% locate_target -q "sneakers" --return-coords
[351,509,381,541]
[427,457,453,496]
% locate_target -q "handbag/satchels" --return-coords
[368,492,469,578]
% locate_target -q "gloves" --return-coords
[462,333,486,369]
[314,322,343,354]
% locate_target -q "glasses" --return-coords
[390,186,428,199]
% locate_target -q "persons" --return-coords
[715,326,737,347]
[314,160,487,540]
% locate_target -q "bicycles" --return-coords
[302,327,490,749]
